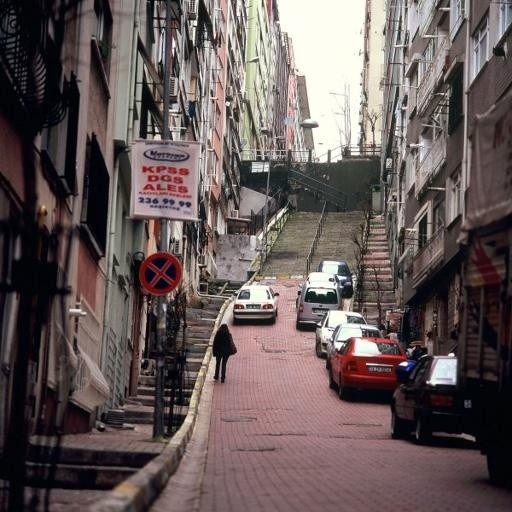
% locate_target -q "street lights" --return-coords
[263,135,286,243]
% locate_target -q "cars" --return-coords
[233,285,279,322]
[296,259,457,444]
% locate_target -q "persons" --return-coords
[211,324,238,381]
[379,324,399,341]
